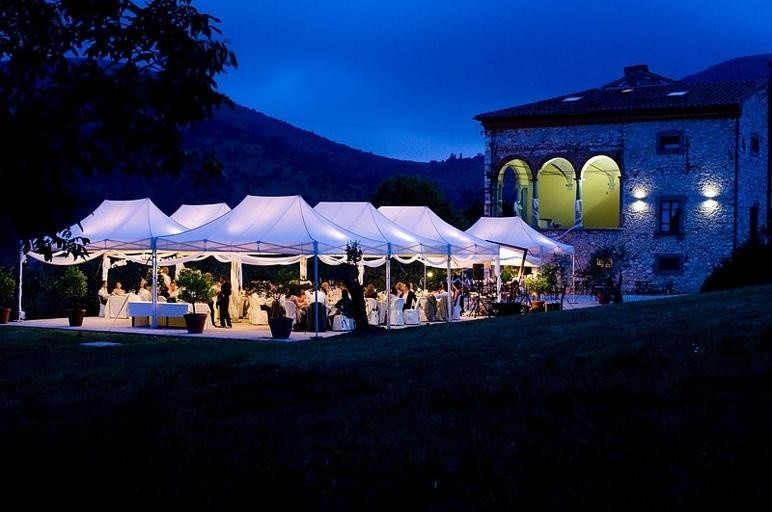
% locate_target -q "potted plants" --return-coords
[54,265,88,326]
[0,270,16,324]
[589,244,630,303]
[523,273,551,312]
[175,267,217,333]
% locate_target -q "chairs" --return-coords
[95,280,465,331]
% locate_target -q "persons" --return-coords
[113,280,126,294]
[389,278,466,322]
[136,268,233,327]
[98,279,111,305]
[256,276,380,328]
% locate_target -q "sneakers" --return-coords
[212,324,233,329]
